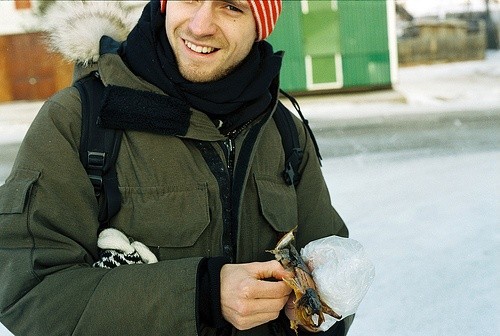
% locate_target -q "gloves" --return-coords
[92,227,158,270]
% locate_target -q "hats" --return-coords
[160,0,282,42]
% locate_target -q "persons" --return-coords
[1,0,356,336]
